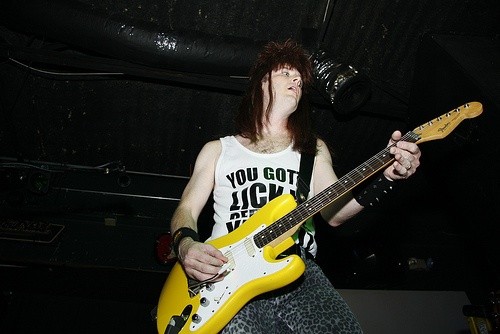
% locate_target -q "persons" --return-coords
[171,38,421,334]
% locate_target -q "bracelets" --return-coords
[173,225,200,259]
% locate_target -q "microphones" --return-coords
[117,160,130,188]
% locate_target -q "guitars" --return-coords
[155,100,483,334]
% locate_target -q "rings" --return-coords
[407,164,412,170]
